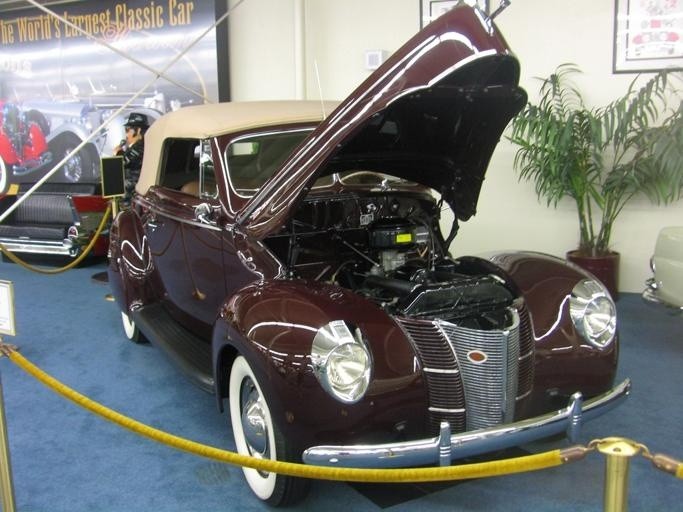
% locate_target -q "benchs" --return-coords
[0,180,110,265]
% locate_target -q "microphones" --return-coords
[120,140,126,147]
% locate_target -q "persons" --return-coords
[112,113,150,211]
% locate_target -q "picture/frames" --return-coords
[419,2,489,43]
[610,1,683,73]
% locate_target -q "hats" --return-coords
[123,113,149,128]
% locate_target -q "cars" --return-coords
[106,0,634,510]
[0,70,182,188]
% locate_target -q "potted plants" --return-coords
[500,62,683,304]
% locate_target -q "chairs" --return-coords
[179,155,267,200]
